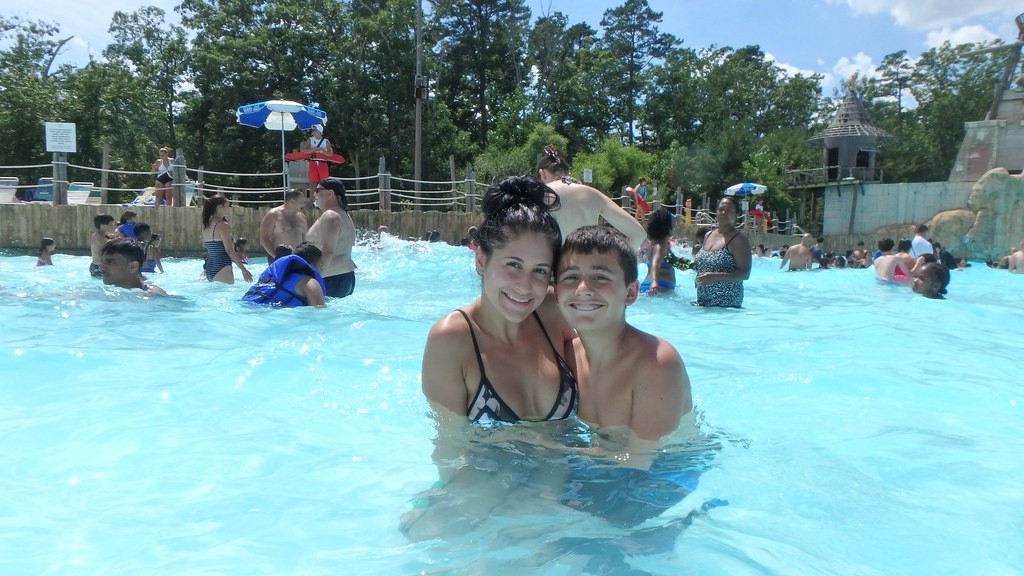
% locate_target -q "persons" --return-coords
[37,238,56,266]
[820,224,968,299]
[634,177,647,222]
[151,148,174,207]
[754,198,764,212]
[554,226,693,440]
[986,240,1024,274]
[421,175,578,422]
[248,177,358,307]
[754,243,788,258]
[780,233,813,270]
[536,146,648,250]
[639,208,676,294]
[201,194,254,284]
[693,195,752,308]
[303,124,333,208]
[425,229,440,242]
[462,225,479,250]
[810,236,825,261]
[90,210,165,293]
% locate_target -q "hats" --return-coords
[312,124,323,135]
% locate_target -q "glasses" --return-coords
[316,188,329,193]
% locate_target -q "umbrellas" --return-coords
[724,181,767,201]
[235,98,328,199]
